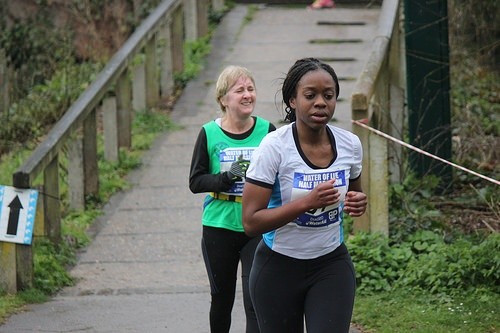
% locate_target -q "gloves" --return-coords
[229,153,250,177]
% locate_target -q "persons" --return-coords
[242,57,368,333]
[188,65,276,333]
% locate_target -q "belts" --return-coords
[209,192,242,202]
